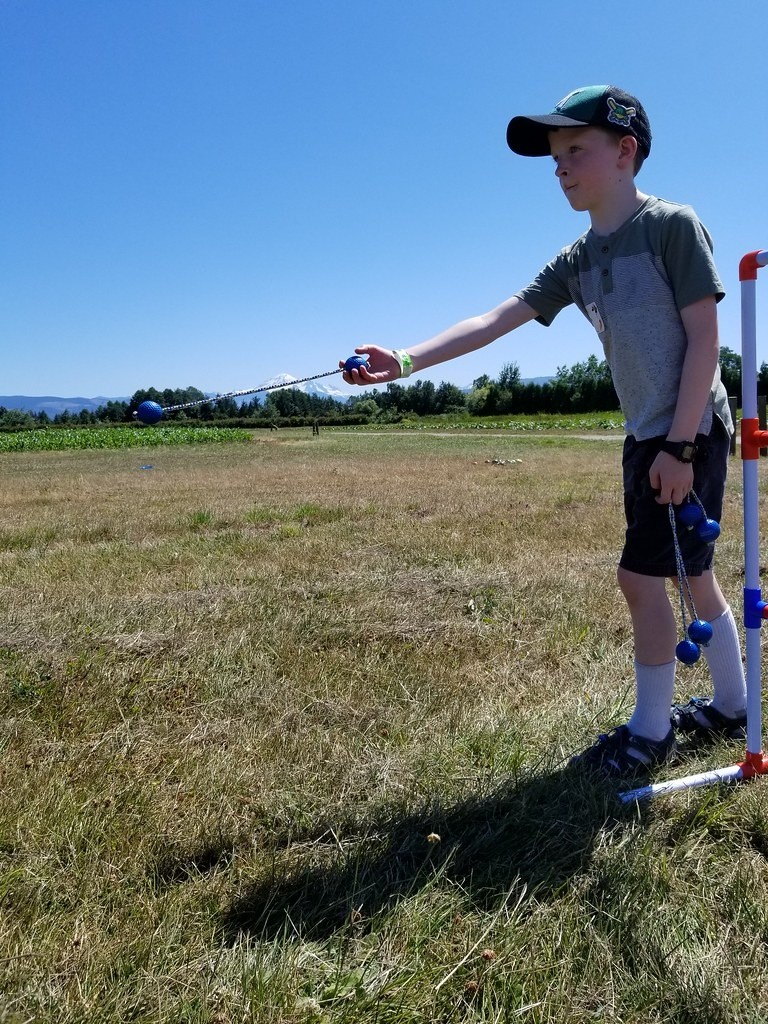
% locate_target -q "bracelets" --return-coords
[392,349,413,378]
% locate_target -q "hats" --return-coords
[505,85,650,158]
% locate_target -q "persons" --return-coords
[339,84,748,783]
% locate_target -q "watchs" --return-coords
[661,441,698,464]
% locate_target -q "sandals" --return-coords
[573,716,677,783]
[669,695,748,752]
